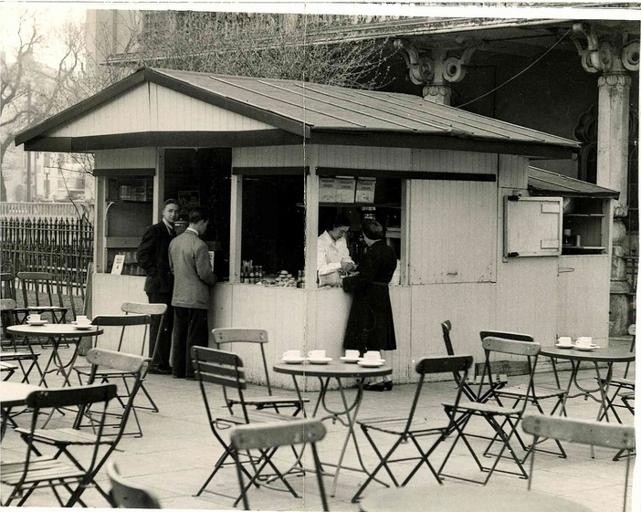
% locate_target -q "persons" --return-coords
[316,212,359,287]
[337,218,398,392]
[134,197,183,376]
[167,206,218,382]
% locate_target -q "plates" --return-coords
[357,359,386,368]
[555,343,574,348]
[72,325,95,330]
[305,358,333,364]
[340,356,359,363]
[25,320,48,325]
[573,347,596,351]
[280,358,304,363]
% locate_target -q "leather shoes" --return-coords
[148,365,172,374]
[362,380,393,391]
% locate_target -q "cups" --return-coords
[307,349,326,359]
[69,316,87,323]
[283,350,300,360]
[363,350,380,362]
[77,319,91,326]
[344,349,361,358]
[557,336,571,346]
[576,336,593,346]
[26,313,40,321]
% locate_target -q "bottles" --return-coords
[239,265,264,283]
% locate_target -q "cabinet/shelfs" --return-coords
[564,213,606,250]
